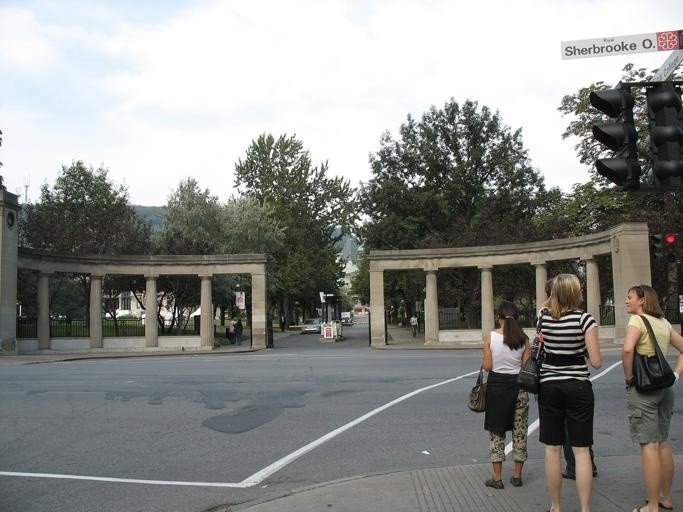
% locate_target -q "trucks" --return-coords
[341,312,351,325]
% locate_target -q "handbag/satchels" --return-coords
[631,313,676,394]
[514,308,546,395]
[466,365,488,412]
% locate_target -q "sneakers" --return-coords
[509,476,523,486]
[483,478,504,489]
[561,469,598,480]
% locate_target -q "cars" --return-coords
[300,319,320,334]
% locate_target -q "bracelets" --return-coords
[624,376,634,381]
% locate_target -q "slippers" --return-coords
[633,498,674,512]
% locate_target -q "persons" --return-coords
[483,273,603,512]
[229,317,244,346]
[409,314,418,338]
[279,313,286,332]
[622,285,683,512]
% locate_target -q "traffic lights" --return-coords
[590,84,635,189]
[651,233,678,264]
